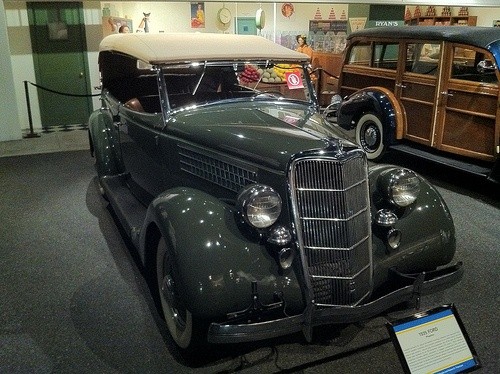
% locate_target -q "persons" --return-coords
[195,3,205,25]
[108,18,147,34]
[295,34,312,59]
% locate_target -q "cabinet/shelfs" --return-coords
[405,14,478,28]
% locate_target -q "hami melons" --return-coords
[257,68,281,82]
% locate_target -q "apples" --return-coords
[239,67,260,84]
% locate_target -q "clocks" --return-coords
[218,8,232,24]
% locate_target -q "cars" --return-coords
[87,32,467,368]
[334,26,499,183]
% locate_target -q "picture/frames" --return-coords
[384,302,482,374]
